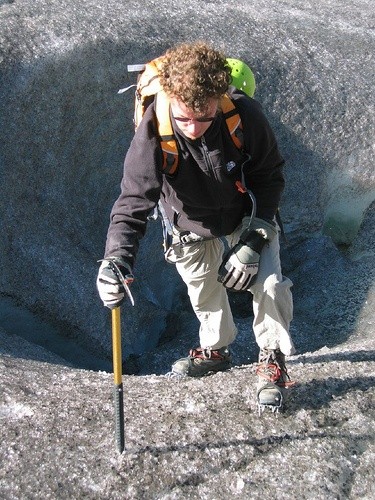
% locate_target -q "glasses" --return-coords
[168,105,217,122]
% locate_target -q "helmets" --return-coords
[224,57,256,100]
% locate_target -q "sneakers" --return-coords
[253,346,295,417]
[163,346,235,384]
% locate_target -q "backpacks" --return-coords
[117,56,257,238]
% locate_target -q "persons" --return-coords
[96,37,296,417]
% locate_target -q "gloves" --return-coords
[217,216,276,293]
[94,256,135,310]
